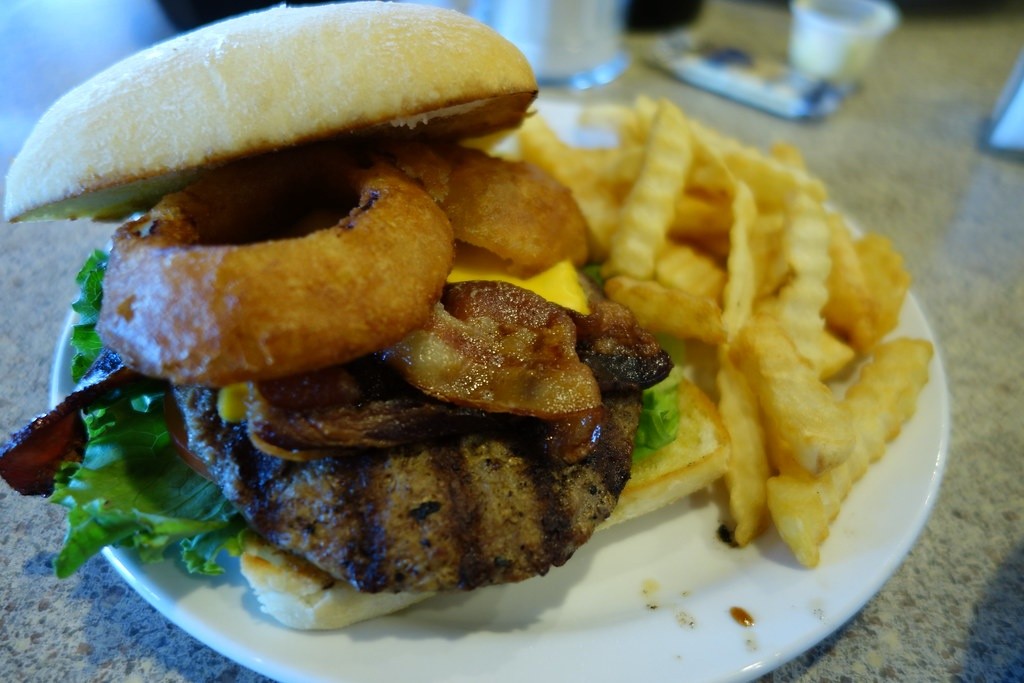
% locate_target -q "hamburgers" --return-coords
[0,4,729,634]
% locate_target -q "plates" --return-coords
[51,97,951,681]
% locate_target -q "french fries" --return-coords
[465,93,936,566]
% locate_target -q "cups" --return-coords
[790,2,897,81]
[461,1,626,91]
